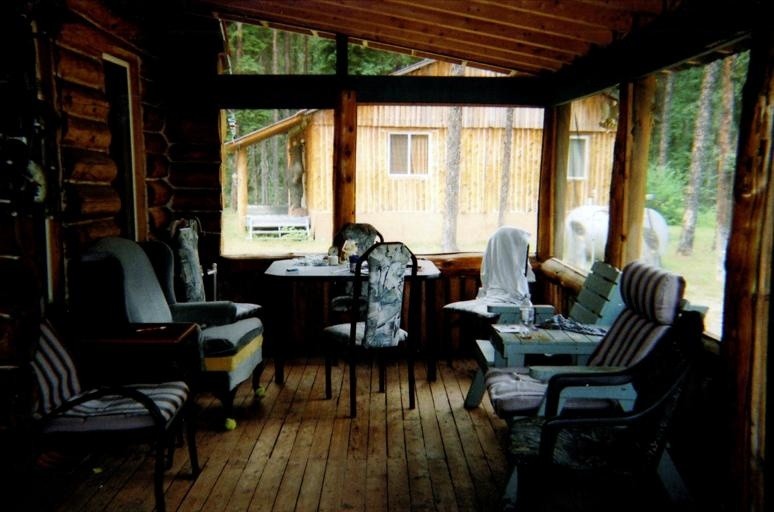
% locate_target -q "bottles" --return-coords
[517,292,534,338]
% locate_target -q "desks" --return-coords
[263,259,441,382]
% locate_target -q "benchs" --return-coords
[247,215,311,240]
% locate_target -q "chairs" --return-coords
[317,222,417,418]
[442,230,530,367]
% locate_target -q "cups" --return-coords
[349,255,360,273]
